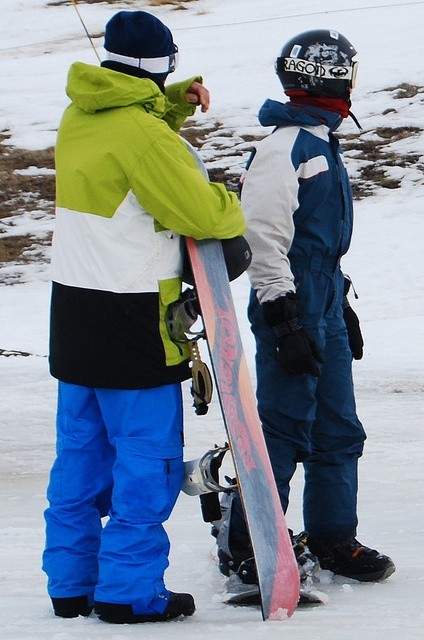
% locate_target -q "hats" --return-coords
[100,12,175,83]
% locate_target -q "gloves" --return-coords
[341,275,363,360]
[261,293,322,378]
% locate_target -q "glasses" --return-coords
[277,56,357,93]
[102,43,177,75]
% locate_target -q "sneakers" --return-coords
[51,595,94,617]
[94,591,194,623]
[217,546,260,584]
[309,535,395,582]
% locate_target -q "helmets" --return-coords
[276,29,358,108]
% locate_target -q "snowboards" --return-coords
[175,134,300,620]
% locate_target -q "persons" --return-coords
[43,10,246,622]
[217,28,395,585]
